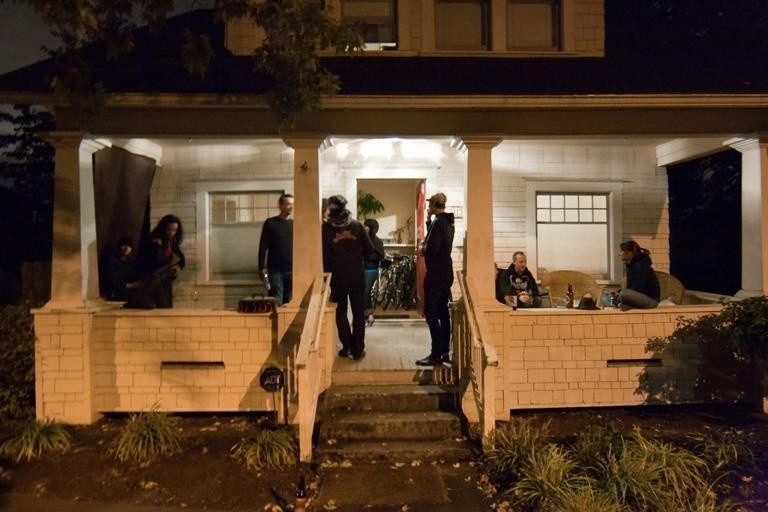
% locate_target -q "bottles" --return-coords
[263,270,271,291]
[567,284,574,307]
[508,286,518,310]
[603,289,622,306]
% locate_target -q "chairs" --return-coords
[541,269,605,308]
[620,272,685,306]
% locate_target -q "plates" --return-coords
[107,301,128,308]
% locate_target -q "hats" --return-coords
[426,193,446,204]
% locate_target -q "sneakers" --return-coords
[339,347,365,360]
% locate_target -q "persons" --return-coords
[415,193,455,367]
[138,214,186,310]
[321,194,384,360]
[104,237,137,303]
[258,194,293,307]
[497,251,542,309]
[618,240,661,312]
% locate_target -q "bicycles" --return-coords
[370,252,419,311]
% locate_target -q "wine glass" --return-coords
[190,289,199,309]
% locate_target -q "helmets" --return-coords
[329,195,346,208]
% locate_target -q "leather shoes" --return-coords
[416,352,449,365]
